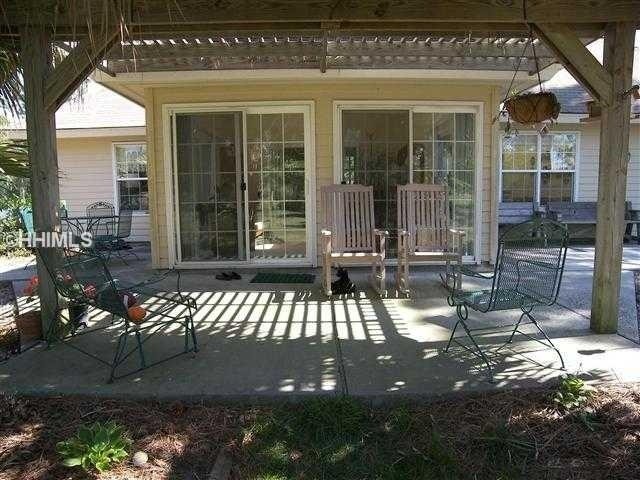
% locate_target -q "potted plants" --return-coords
[503,92,559,138]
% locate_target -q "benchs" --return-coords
[537,200,639,247]
[38,246,198,383]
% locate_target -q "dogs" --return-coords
[331,267,357,295]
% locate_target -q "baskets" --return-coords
[503,91,561,124]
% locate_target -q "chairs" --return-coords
[318,182,570,385]
[19,202,142,269]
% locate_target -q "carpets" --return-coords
[250,271,316,284]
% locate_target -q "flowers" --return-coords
[18,276,42,311]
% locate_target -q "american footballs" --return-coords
[128,306,146,320]
[94,291,136,310]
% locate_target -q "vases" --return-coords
[17,311,42,336]
